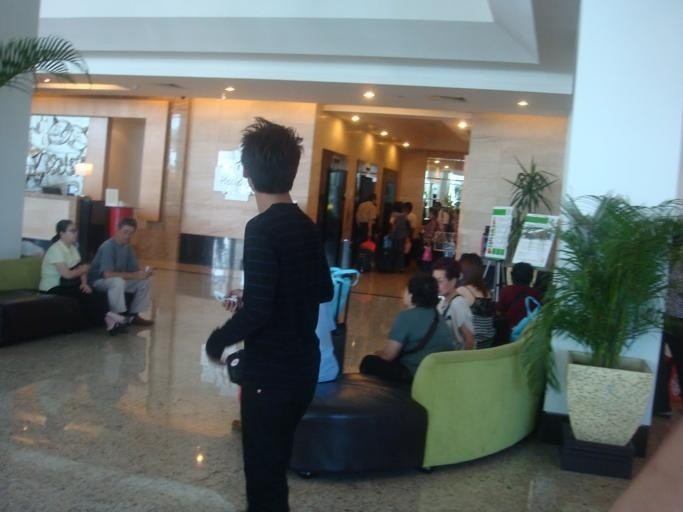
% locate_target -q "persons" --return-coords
[348,189,457,276]
[426,254,474,350]
[36,219,137,336]
[226,240,352,433]
[359,274,454,387]
[492,262,543,329]
[87,219,156,327]
[455,252,497,347]
[203,116,333,512]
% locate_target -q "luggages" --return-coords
[379,246,400,273]
[359,241,377,272]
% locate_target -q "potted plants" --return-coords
[510,190,681,480]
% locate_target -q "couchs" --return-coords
[295,328,546,479]
[0,257,135,351]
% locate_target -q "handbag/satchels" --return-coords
[59,270,83,293]
[359,354,405,379]
[512,296,543,340]
[422,245,433,262]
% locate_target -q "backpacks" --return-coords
[391,212,409,238]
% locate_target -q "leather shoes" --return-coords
[133,315,154,326]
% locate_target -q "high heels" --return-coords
[108,315,137,337]
